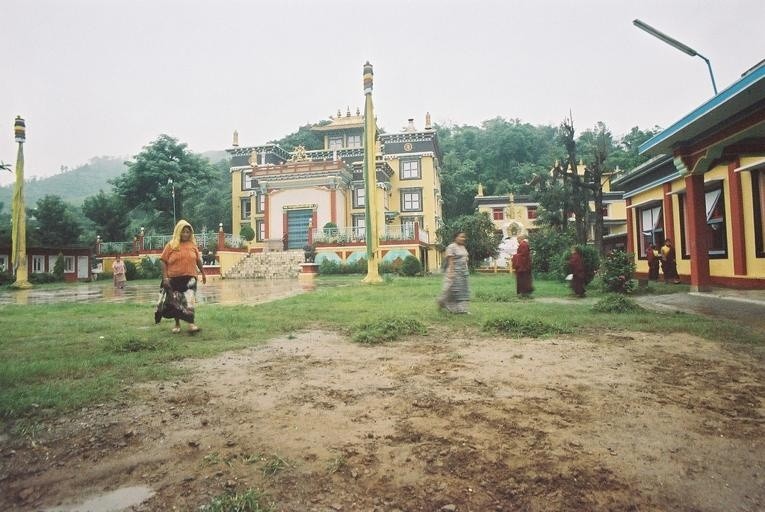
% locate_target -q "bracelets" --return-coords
[163,277,168,280]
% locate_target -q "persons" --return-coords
[435,230,473,315]
[569,246,587,299]
[647,242,660,281]
[660,239,681,284]
[157,219,207,333]
[112,256,128,289]
[510,235,536,296]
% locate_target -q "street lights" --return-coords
[167,176,177,226]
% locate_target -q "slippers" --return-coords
[172,327,181,333]
[189,327,200,333]
